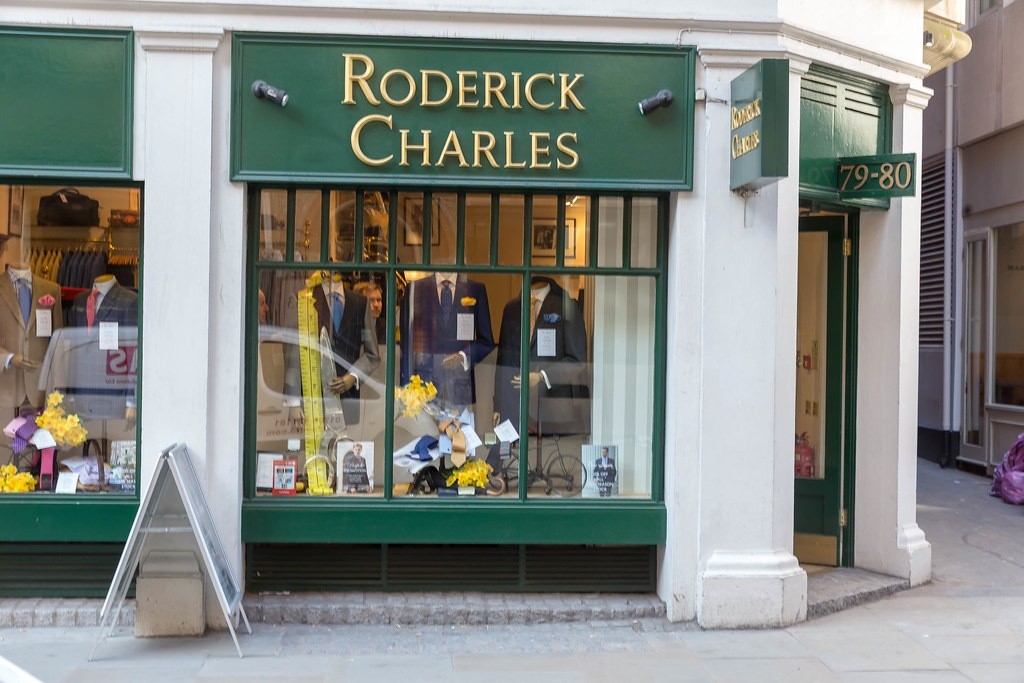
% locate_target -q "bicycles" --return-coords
[486,432,587,496]
[0,444,57,477]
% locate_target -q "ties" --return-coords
[87,288,101,328]
[528,295,536,342]
[406,434,440,462]
[440,280,452,331]
[17,277,33,328]
[331,291,345,335]
[11,414,40,455]
[440,417,467,468]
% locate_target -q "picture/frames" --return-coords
[404,197,441,246]
[9,185,25,236]
[531,217,577,258]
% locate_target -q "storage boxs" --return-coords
[110,210,139,227]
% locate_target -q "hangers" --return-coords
[29,236,139,266]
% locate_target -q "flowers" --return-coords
[395,374,437,417]
[0,461,37,494]
[446,458,493,490]
[35,390,88,447]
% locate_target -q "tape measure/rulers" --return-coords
[297,289,335,496]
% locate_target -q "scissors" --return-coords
[303,326,356,490]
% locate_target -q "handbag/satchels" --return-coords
[37,187,100,226]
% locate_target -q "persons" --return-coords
[0,262,65,408]
[346,443,373,494]
[593,447,616,497]
[67,274,138,433]
[258,287,269,326]
[282,273,382,434]
[492,275,588,437]
[399,271,496,406]
[352,282,383,318]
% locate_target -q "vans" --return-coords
[37,322,440,489]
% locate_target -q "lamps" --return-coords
[638,89,675,116]
[251,79,288,107]
